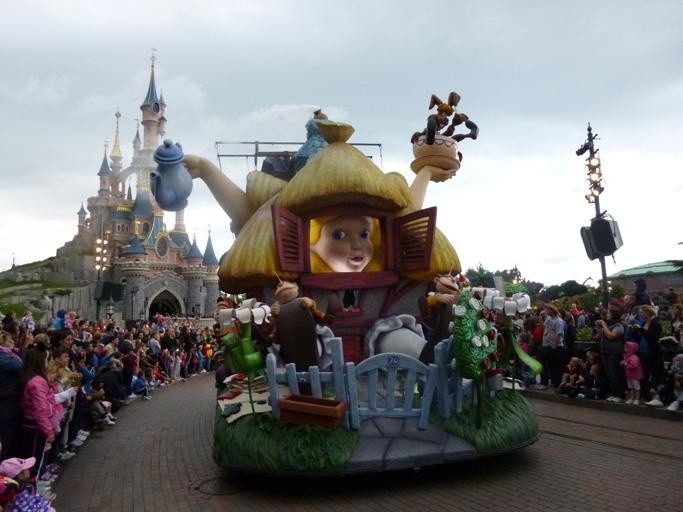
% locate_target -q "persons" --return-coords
[415,278,463,402]
[1,308,221,512]
[179,155,462,278]
[270,282,325,397]
[474,279,683,411]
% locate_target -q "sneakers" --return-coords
[143,396,152,401]
[66,429,90,452]
[56,451,75,461]
[41,462,64,482]
[37,480,57,501]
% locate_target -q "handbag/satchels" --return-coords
[86,411,104,431]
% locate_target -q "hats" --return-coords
[0,456,37,479]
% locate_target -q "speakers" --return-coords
[93,280,124,302]
[580,217,623,261]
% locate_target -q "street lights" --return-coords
[575,122,624,308]
[95,238,126,321]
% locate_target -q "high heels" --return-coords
[104,413,117,425]
